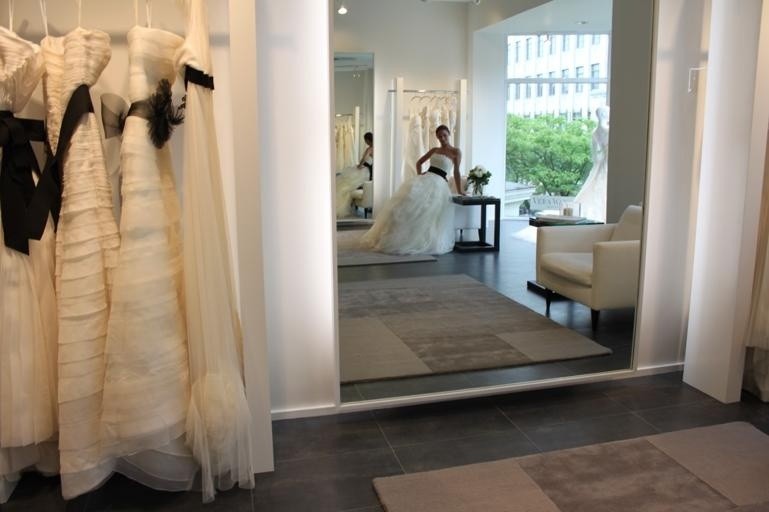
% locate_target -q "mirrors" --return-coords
[331,52,377,227]
[327,1,661,414]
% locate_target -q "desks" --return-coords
[527,213,605,303]
[451,196,502,254]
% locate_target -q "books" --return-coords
[535,214,587,224]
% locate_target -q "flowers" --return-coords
[466,165,493,194]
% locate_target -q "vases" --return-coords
[473,184,483,198]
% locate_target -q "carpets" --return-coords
[336,229,438,265]
[337,271,615,384]
[367,417,769,511]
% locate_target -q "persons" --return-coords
[359,124,473,256]
[335,131,374,217]
[568,104,610,225]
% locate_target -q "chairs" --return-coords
[448,175,482,235]
[352,180,373,220]
[535,204,645,336]
